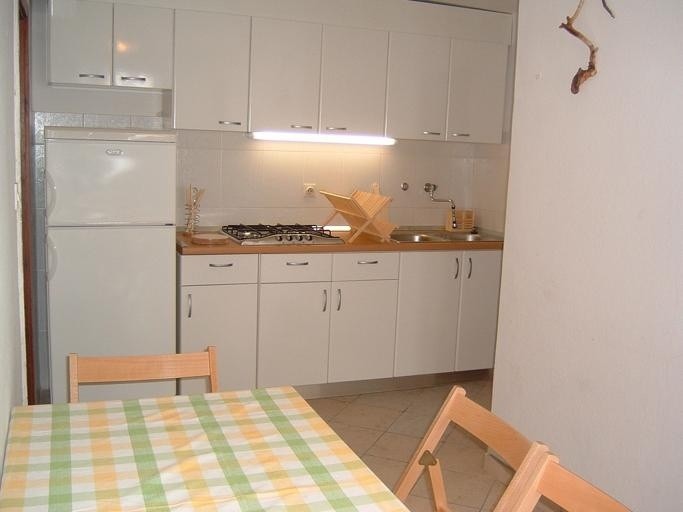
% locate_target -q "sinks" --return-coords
[387,232,503,242]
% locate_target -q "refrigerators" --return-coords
[44,126,177,405]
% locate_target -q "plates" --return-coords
[192,233,229,244]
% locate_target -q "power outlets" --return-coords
[304,183,316,198]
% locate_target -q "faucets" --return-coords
[423,182,457,229]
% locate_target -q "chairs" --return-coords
[511,433,640,512]
[67,345,220,402]
[393,384,547,511]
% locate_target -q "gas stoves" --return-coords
[221,223,340,240]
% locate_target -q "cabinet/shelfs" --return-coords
[258,253,398,388]
[383,33,513,144]
[49,1,171,88]
[162,9,249,131]
[180,251,260,395]
[394,251,502,375]
[247,16,390,135]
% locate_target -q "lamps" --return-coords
[246,131,401,146]
